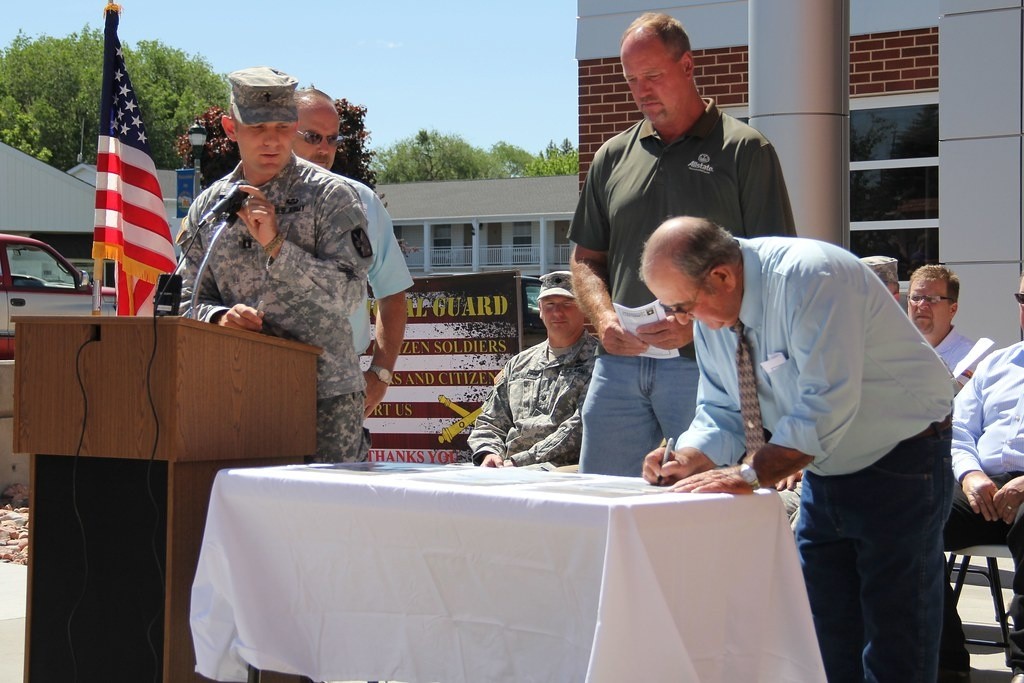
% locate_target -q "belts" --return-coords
[909,413,952,438]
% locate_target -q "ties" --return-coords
[729,318,766,456]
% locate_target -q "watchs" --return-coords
[369,364,392,385]
[738,462,761,491]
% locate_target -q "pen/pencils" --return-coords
[257,299,264,319]
[658,437,674,484]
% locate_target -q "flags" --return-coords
[91,2,177,319]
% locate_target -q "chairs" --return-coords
[947,545,1012,670]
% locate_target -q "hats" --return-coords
[228,67,298,125]
[859,256,898,283]
[537,271,576,300]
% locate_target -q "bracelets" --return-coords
[263,232,284,253]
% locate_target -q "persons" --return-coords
[775,255,900,535]
[907,264,995,389]
[937,271,1024,683]
[639,215,955,683]
[467,271,599,468]
[175,67,374,464]
[566,12,798,478]
[290,88,414,421]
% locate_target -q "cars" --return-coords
[519,276,547,337]
[0,234,119,360]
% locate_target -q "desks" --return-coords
[189,461,828,683]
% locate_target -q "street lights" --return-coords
[187,119,206,201]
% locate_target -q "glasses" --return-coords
[1014,293,1024,304]
[659,271,709,313]
[296,130,344,146]
[908,295,953,304]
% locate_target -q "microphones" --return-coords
[198,179,250,228]
